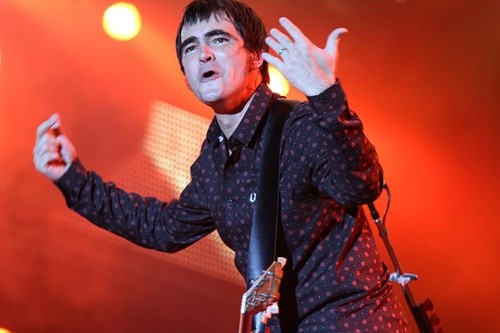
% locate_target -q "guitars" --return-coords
[238,257,287,332]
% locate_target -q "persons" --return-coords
[33,0,412,333]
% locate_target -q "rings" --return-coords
[279,47,287,56]
